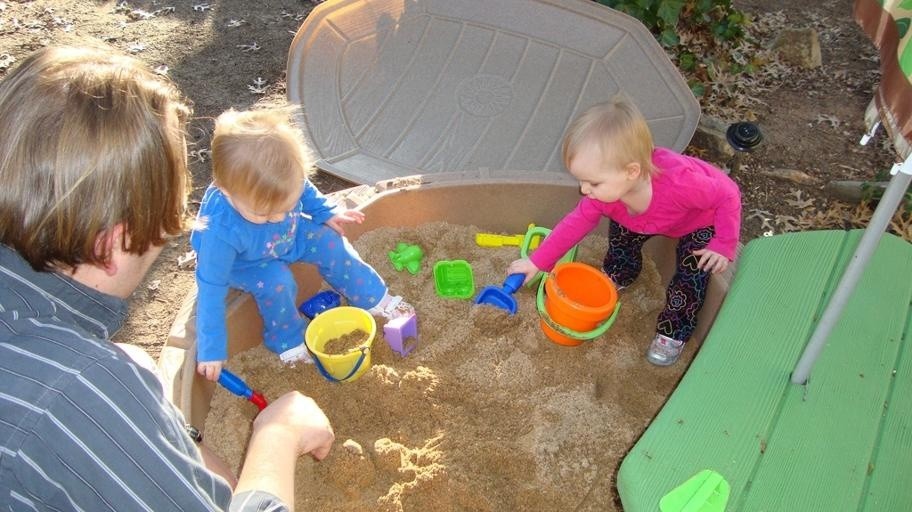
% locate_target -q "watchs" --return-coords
[183,423,203,443]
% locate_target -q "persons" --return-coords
[1,44,335,512]
[505,92,745,368]
[189,101,415,385]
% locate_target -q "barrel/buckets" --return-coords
[537,262,621,347]
[304,307,377,382]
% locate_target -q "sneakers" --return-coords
[280,344,315,372]
[368,289,415,322]
[645,332,686,368]
